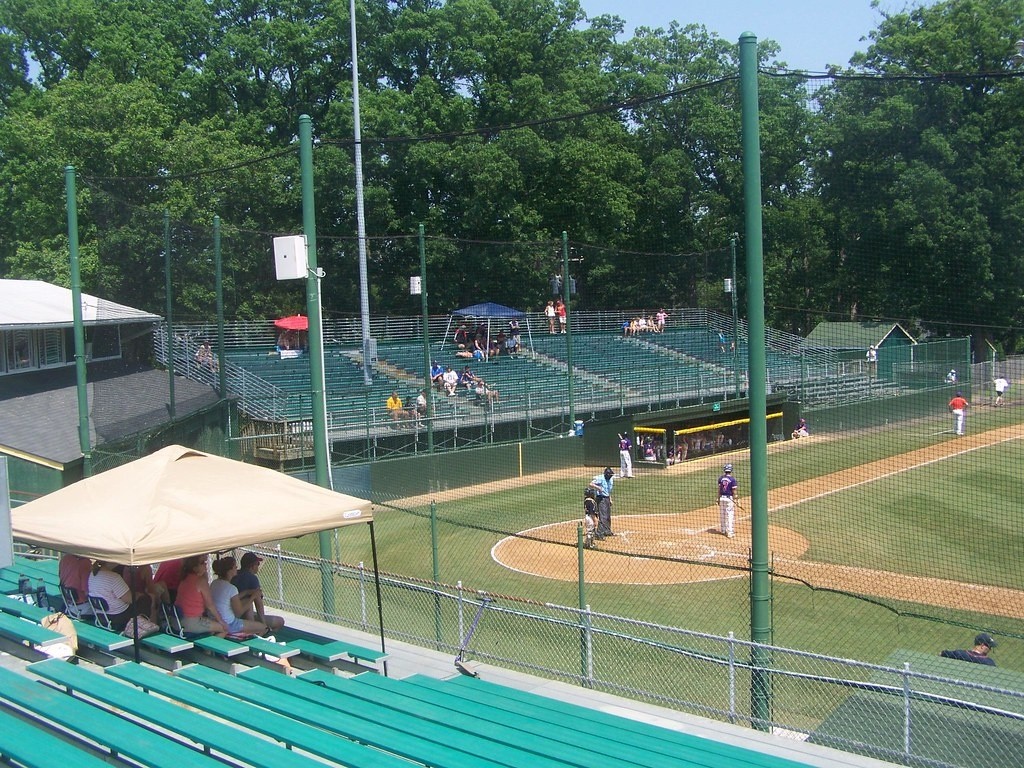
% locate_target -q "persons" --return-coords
[793,419,809,439]
[59,552,285,661]
[545,300,567,334]
[639,417,745,464]
[949,392,968,435]
[866,345,876,360]
[430,360,498,403]
[619,432,635,478]
[584,468,615,547]
[386,389,434,429]
[454,317,520,362]
[621,307,668,336]
[993,376,1008,407]
[944,369,957,384]
[195,341,214,372]
[277,329,309,350]
[942,633,998,666]
[717,464,738,538]
[718,329,734,353]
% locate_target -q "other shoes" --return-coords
[598,537,606,541]
[610,534,617,537]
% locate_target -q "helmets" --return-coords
[623,432,629,437]
[603,468,615,475]
[723,464,734,473]
[584,487,594,494]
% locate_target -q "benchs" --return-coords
[174,328,833,436]
[0,554,819,768]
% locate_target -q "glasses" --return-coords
[986,645,992,650]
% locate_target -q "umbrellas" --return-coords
[274,314,308,347]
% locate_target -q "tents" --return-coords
[441,303,535,363]
[10,444,387,677]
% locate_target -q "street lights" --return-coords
[726,231,742,399]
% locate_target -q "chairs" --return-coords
[156,603,215,658]
[58,586,94,622]
[87,595,127,631]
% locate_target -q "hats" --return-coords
[974,633,998,647]
[869,345,874,349]
[241,552,263,566]
[955,391,962,396]
[800,418,806,423]
[950,370,955,372]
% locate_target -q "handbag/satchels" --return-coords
[41,611,79,651]
[124,613,160,640]
